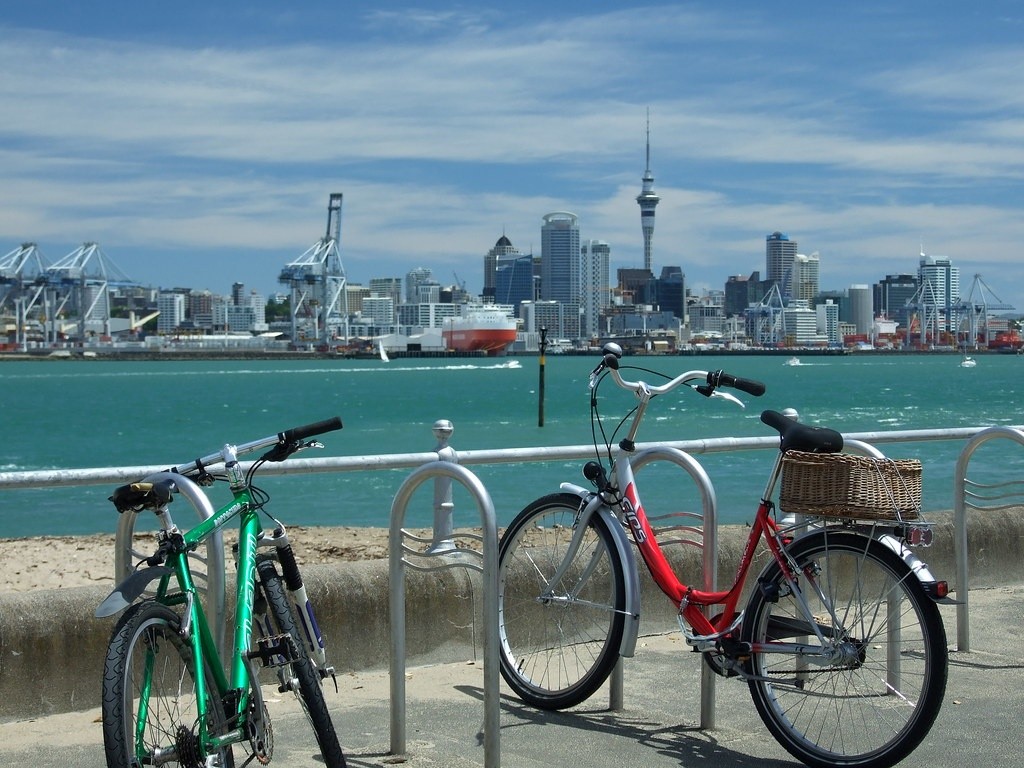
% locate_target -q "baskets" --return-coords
[779,450,923,520]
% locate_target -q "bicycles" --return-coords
[94,416,349,767]
[498,341,965,767]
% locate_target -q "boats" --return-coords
[443,310,517,357]
